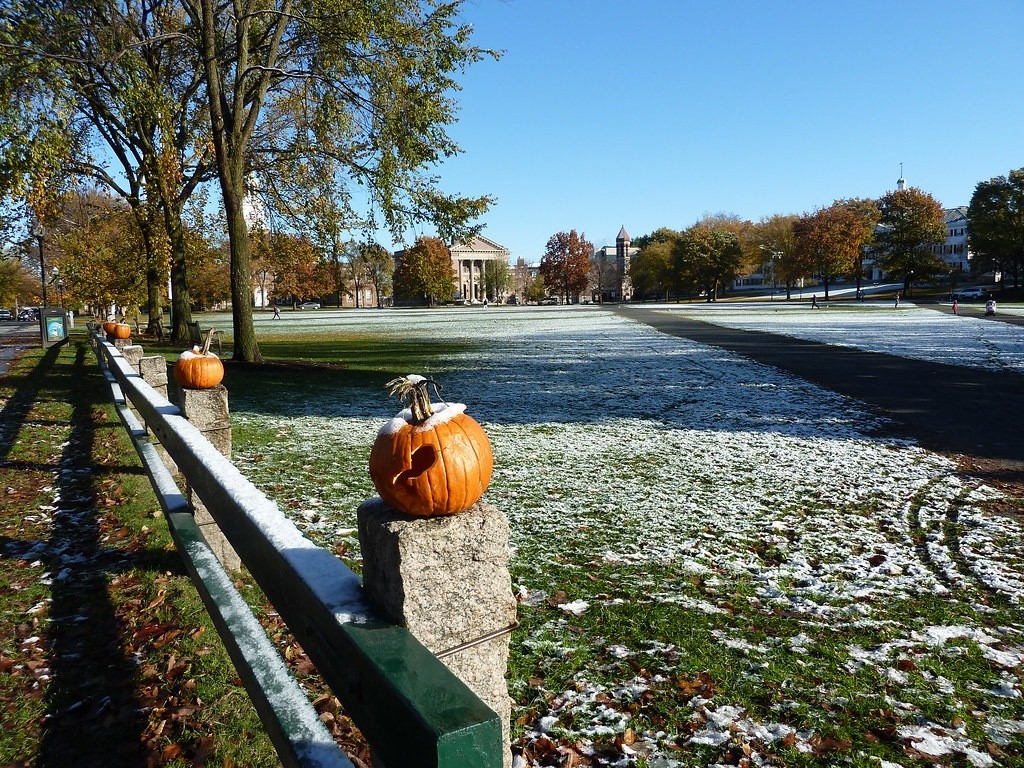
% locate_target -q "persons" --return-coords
[857,289,865,302]
[484,299,488,310]
[894,291,900,308]
[952,300,957,314]
[988,294,995,301]
[811,294,819,309]
[272,305,280,320]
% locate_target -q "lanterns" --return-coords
[368,405,493,517]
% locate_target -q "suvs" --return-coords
[540,298,557,305]
[954,288,988,299]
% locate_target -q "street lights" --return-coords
[57,279,64,308]
[33,221,47,309]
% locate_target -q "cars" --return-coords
[0,310,11,321]
[297,302,320,310]
[581,300,593,305]
[18,310,36,321]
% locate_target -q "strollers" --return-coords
[985,300,997,315]
